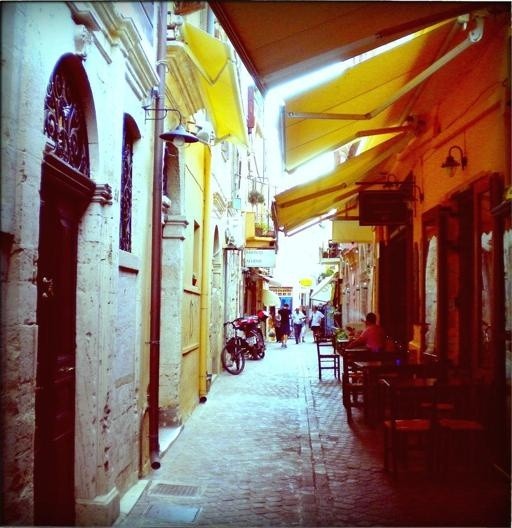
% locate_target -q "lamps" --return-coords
[141,105,199,147]
[441,144,467,178]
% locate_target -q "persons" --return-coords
[344,312,385,359]
[258,303,326,348]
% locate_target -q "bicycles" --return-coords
[221,309,269,374]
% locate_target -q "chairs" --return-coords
[315,334,496,490]
